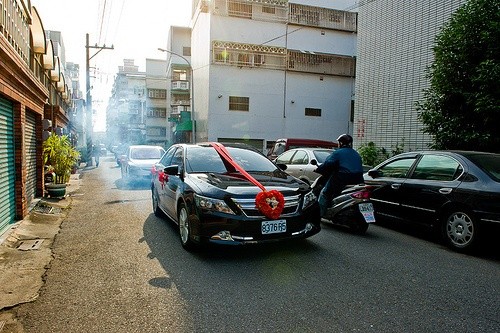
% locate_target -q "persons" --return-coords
[314,133,364,223]
[114,143,125,164]
[93,140,101,167]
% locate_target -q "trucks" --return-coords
[268,138,338,161]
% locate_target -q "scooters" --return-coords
[310,158,376,234]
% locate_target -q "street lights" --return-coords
[157,47,195,144]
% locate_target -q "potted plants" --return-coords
[42,133,81,200]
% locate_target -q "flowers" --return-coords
[255,190,285,219]
[159,171,168,190]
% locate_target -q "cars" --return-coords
[99,143,167,187]
[273,147,335,185]
[151,143,323,251]
[363,151,500,261]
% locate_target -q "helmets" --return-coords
[336,133,354,144]
